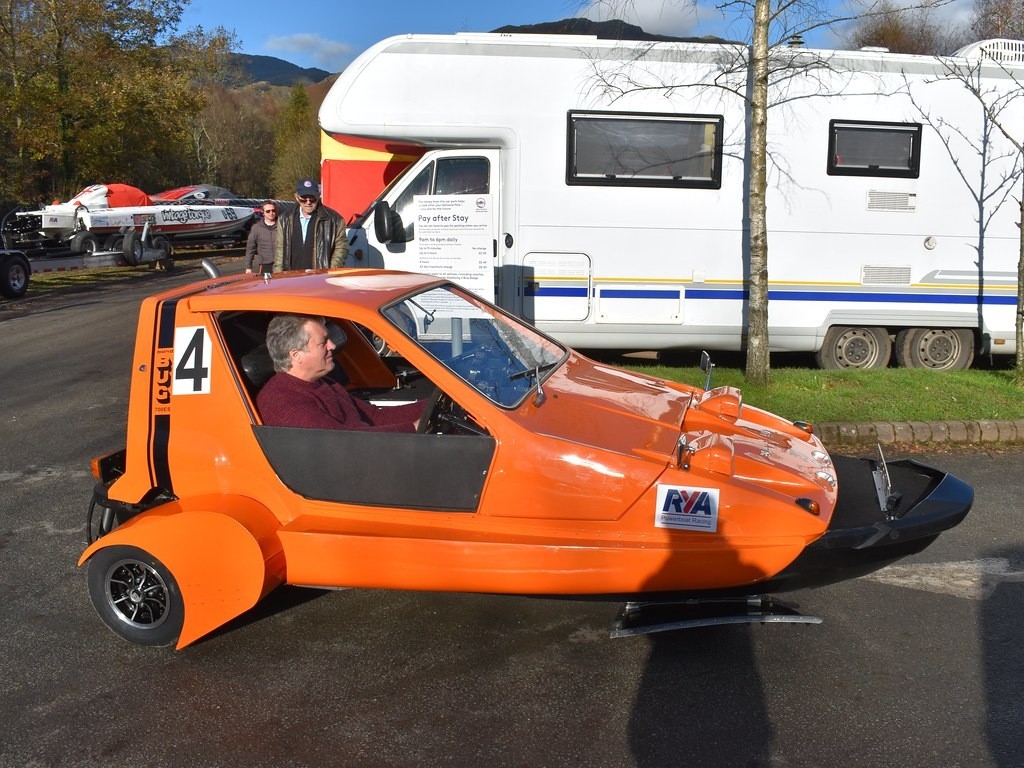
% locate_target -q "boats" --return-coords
[14,182,264,241]
[149,184,296,228]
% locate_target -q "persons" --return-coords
[243,200,279,275]
[272,178,349,273]
[251,312,446,434]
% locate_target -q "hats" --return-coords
[295,179,320,197]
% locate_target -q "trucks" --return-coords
[312,30,1024,373]
[316,121,717,230]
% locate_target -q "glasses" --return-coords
[264,209,276,213]
[297,195,317,203]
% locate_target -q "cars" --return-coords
[76,258,978,654]
